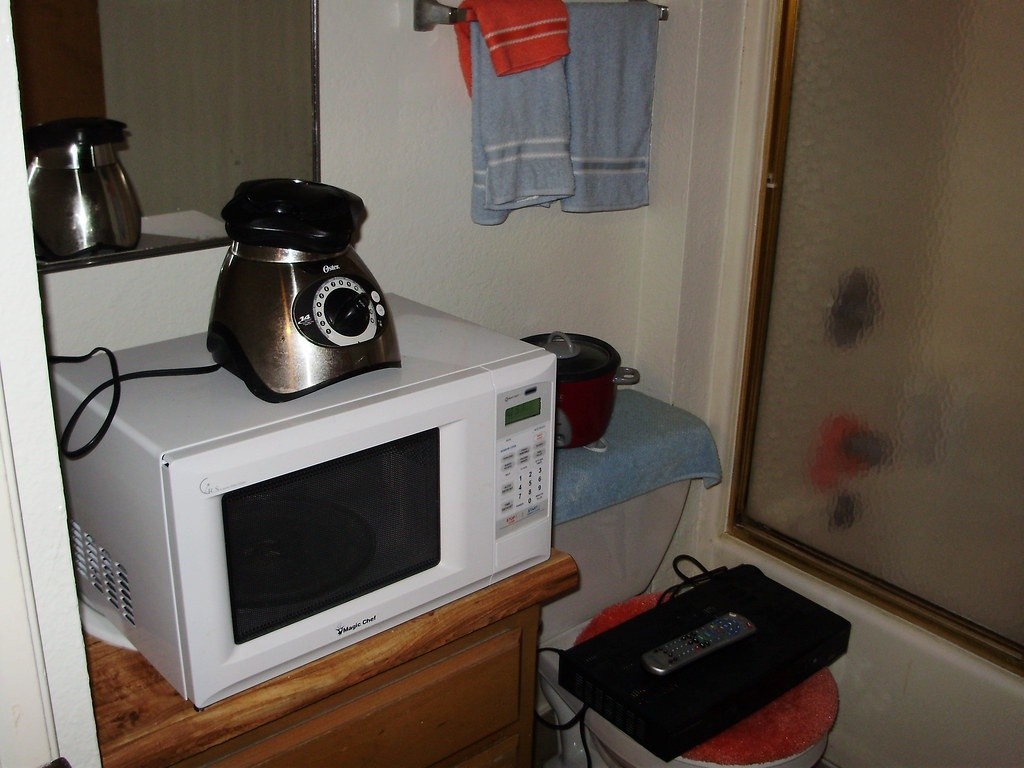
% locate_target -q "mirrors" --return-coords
[10,0,322,273]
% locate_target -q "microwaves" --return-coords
[51,292,553,710]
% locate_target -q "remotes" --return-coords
[644,612,757,675]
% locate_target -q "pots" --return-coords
[520,331,640,452]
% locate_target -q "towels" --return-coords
[453,0,571,99]
[469,22,577,226]
[559,1,660,214]
[553,387,723,530]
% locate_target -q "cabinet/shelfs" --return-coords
[82,549,581,768]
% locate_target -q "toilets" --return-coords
[537,388,841,768]
